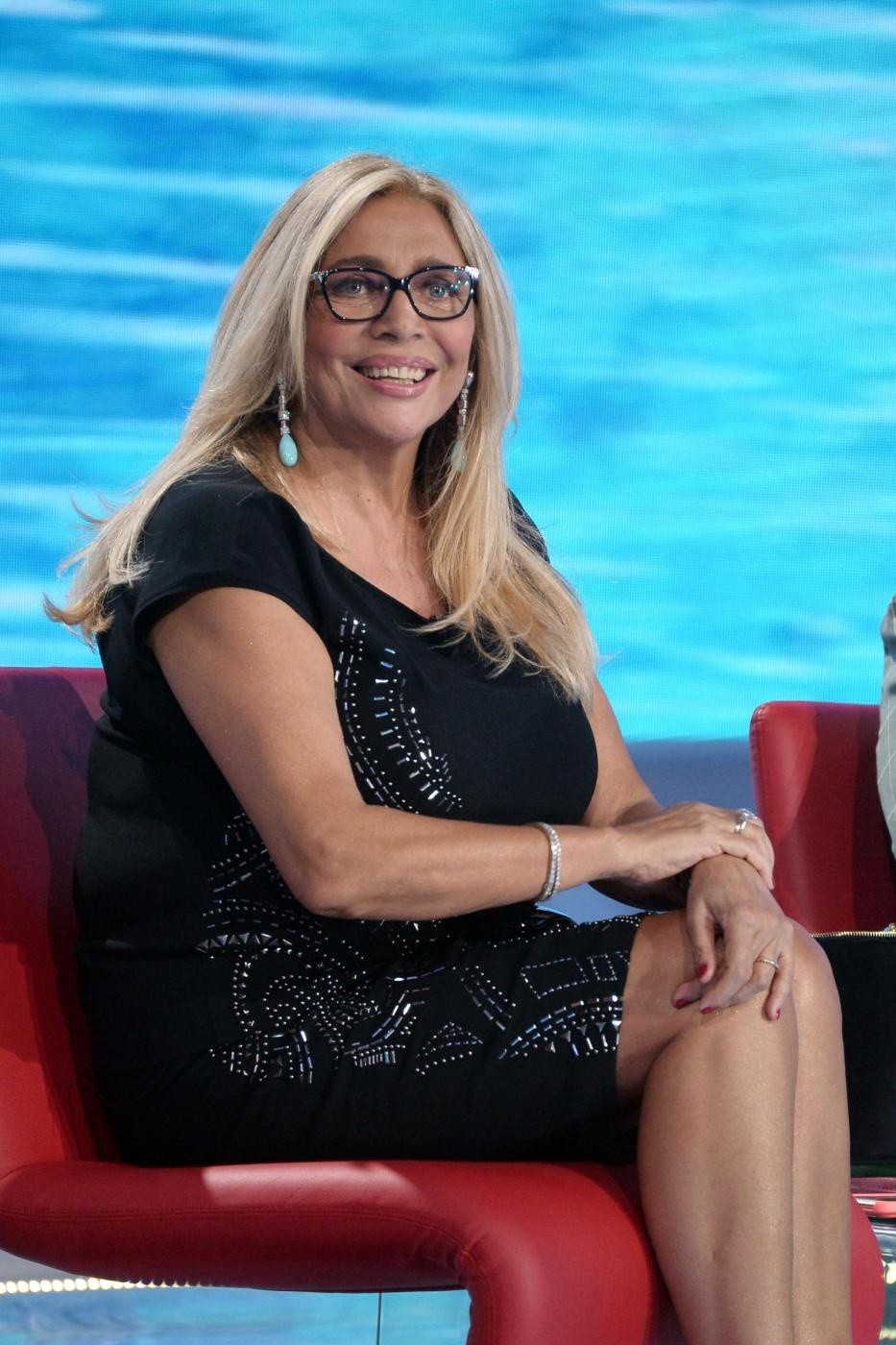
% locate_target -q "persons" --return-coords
[43,154,852,1345]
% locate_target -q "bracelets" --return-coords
[525,822,563,901]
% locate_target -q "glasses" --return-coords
[309,266,479,323]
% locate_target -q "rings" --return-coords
[734,806,759,835]
[756,959,778,970]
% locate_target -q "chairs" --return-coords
[0,666,887,1344]
[749,699,896,1231]
